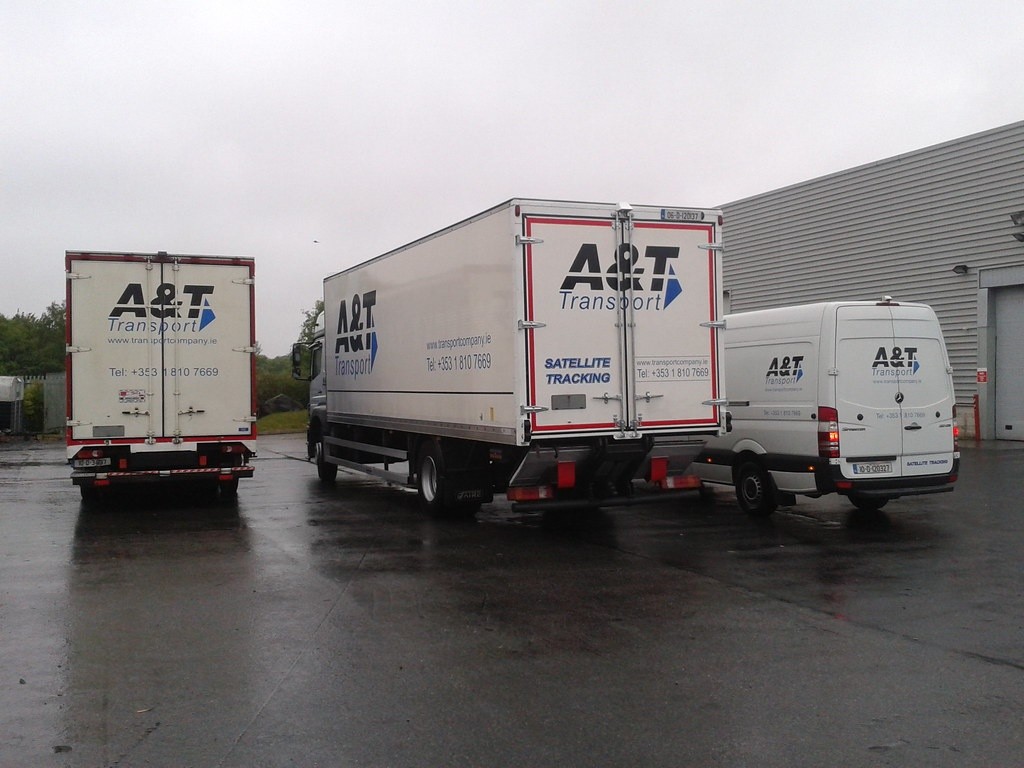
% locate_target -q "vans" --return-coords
[680,296,961,520]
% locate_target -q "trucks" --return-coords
[292,196,750,521]
[64,250,258,503]
